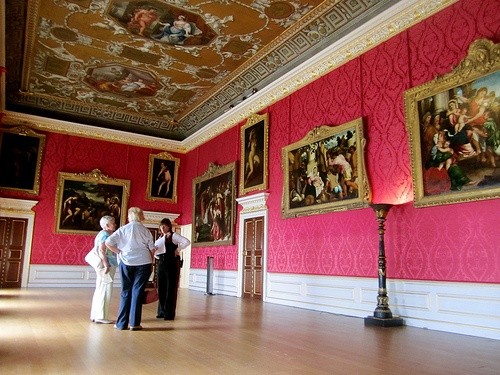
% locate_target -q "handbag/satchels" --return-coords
[84,231,109,269]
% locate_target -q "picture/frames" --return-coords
[401,38,500,209]
[240,112,269,196]
[190,162,237,248]
[280,118,373,219]
[145,152,181,206]
[0,125,46,197]
[53,168,131,237]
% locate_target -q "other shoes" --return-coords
[156,315,174,320]
[91,319,113,324]
[113,324,119,328]
[130,325,142,331]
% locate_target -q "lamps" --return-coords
[364,202,406,328]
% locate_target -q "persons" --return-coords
[154,218,191,321]
[105,207,159,331]
[90,216,119,324]
